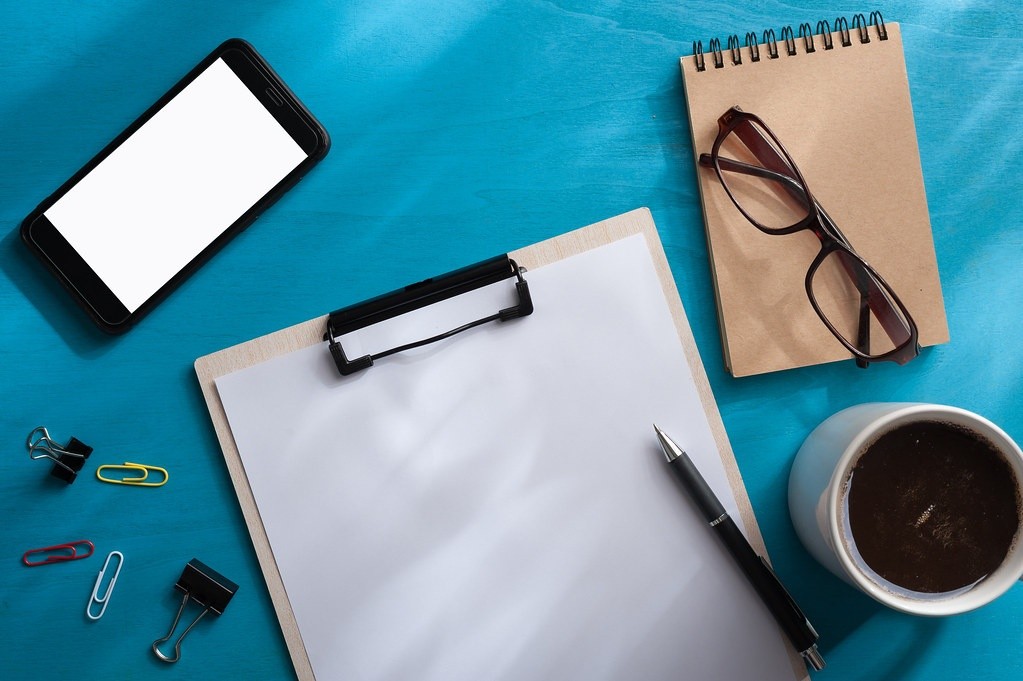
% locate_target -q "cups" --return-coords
[786,402,1022,618]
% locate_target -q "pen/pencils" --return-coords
[653,424,827,673]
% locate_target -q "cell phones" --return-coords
[22,36,330,334]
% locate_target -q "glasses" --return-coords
[699,106,922,368]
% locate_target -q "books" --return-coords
[680,11,949,378]
